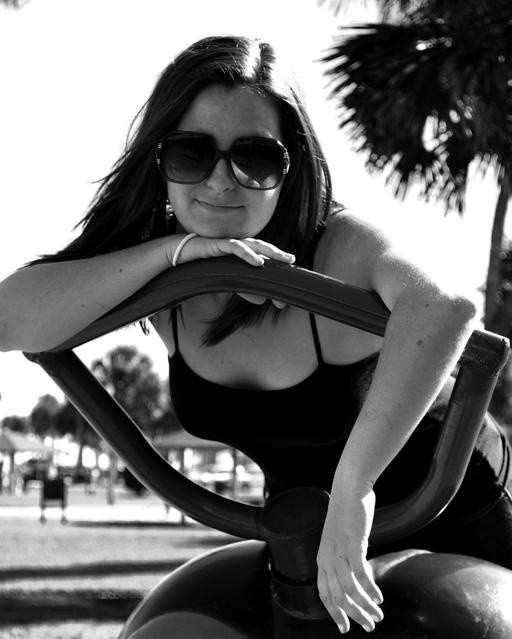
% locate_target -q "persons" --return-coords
[0,36,512,639]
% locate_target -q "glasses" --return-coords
[155,130,292,191]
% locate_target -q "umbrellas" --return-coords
[0,424,70,483]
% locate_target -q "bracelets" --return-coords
[172,233,198,265]
